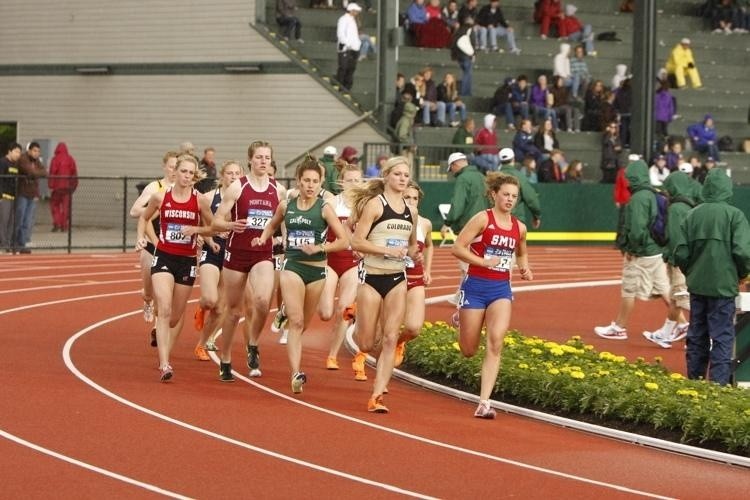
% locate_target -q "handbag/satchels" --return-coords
[456,28,474,56]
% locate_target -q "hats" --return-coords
[324,146,337,155]
[499,148,514,161]
[679,163,693,173]
[347,3,362,12]
[342,146,357,158]
[447,152,466,172]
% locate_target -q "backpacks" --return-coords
[649,193,668,247]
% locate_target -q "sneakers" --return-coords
[206,339,218,351]
[160,365,173,381]
[452,310,460,327]
[249,369,261,377]
[352,351,367,380]
[219,362,234,382]
[394,343,404,366]
[343,304,356,321]
[447,296,458,305]
[473,401,496,419]
[594,323,628,340]
[279,329,288,344]
[144,297,154,323]
[326,356,339,369]
[671,324,689,341]
[271,304,287,333]
[643,330,672,348]
[195,304,204,331]
[368,394,389,413]
[292,373,306,393]
[194,345,210,360]
[245,341,260,369]
[150,327,157,346]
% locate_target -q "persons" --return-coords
[275,0,304,44]
[180,140,203,192]
[197,146,222,194]
[348,154,425,414]
[648,0,750,186]
[316,146,342,196]
[391,0,652,183]
[640,169,713,353]
[363,154,389,178]
[210,139,289,383]
[133,154,221,382]
[440,152,496,310]
[329,2,362,94]
[451,170,534,420]
[193,159,244,361]
[12,141,49,255]
[129,150,178,348]
[334,144,358,194]
[613,153,643,250]
[371,178,434,395]
[594,160,690,341]
[264,156,288,203]
[326,0,350,11]
[249,153,351,396]
[339,177,387,383]
[270,160,336,344]
[0,143,23,255]
[352,15,378,61]
[309,0,326,9]
[671,167,750,388]
[49,142,79,233]
[350,0,377,15]
[496,148,541,282]
[310,156,364,370]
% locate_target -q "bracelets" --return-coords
[319,243,326,253]
[226,222,230,232]
